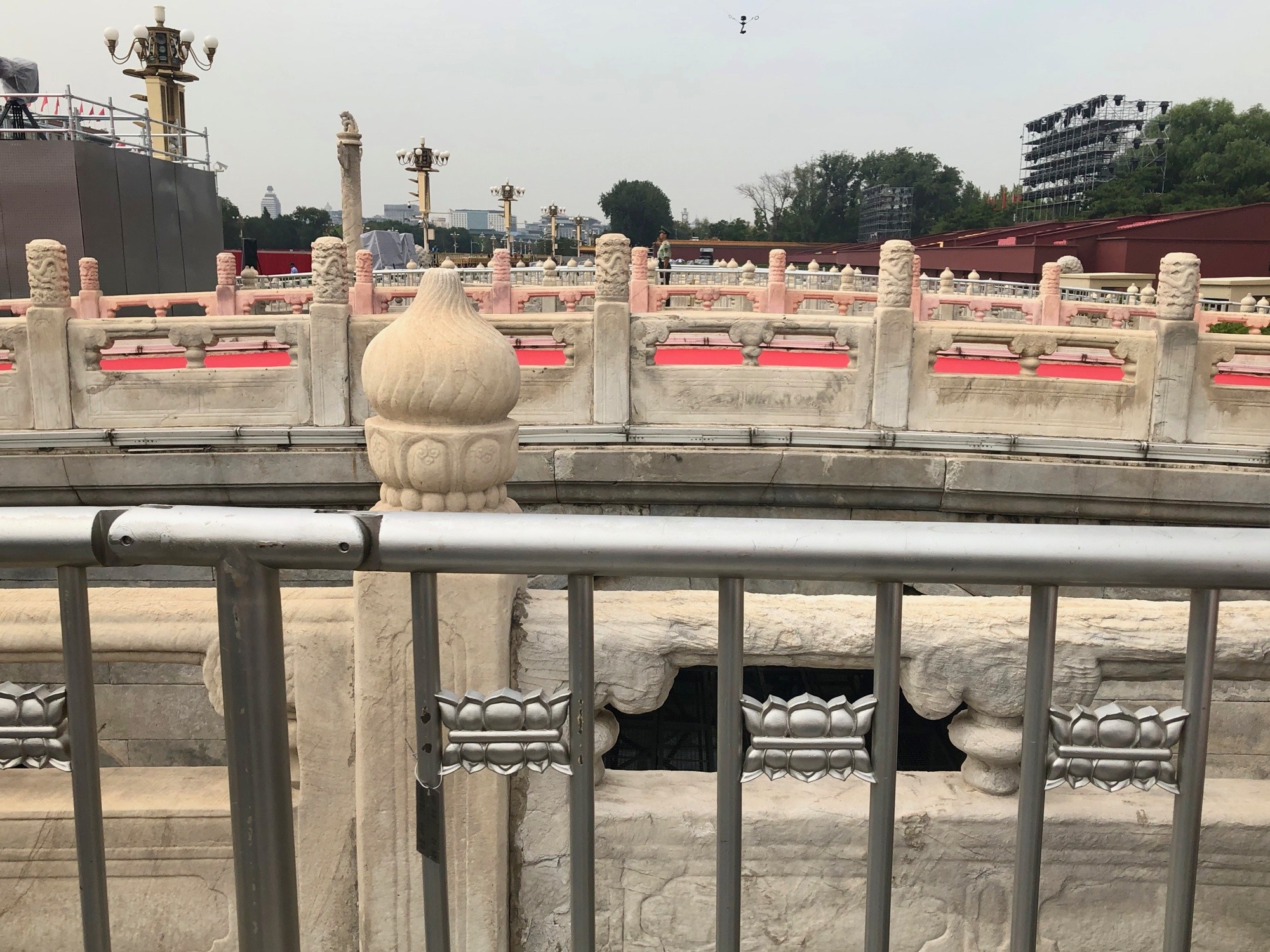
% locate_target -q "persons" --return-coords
[657,225,672,307]
[290,262,298,273]
[432,244,439,252]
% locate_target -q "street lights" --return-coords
[490,178,525,254]
[568,212,589,256]
[527,238,539,255]
[500,235,508,249]
[479,232,486,252]
[101,5,219,162]
[490,234,498,254]
[518,237,524,255]
[541,200,566,257]
[450,231,460,253]
[395,136,451,253]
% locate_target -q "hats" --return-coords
[658,225,671,234]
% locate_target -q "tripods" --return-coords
[0,98,47,140]
